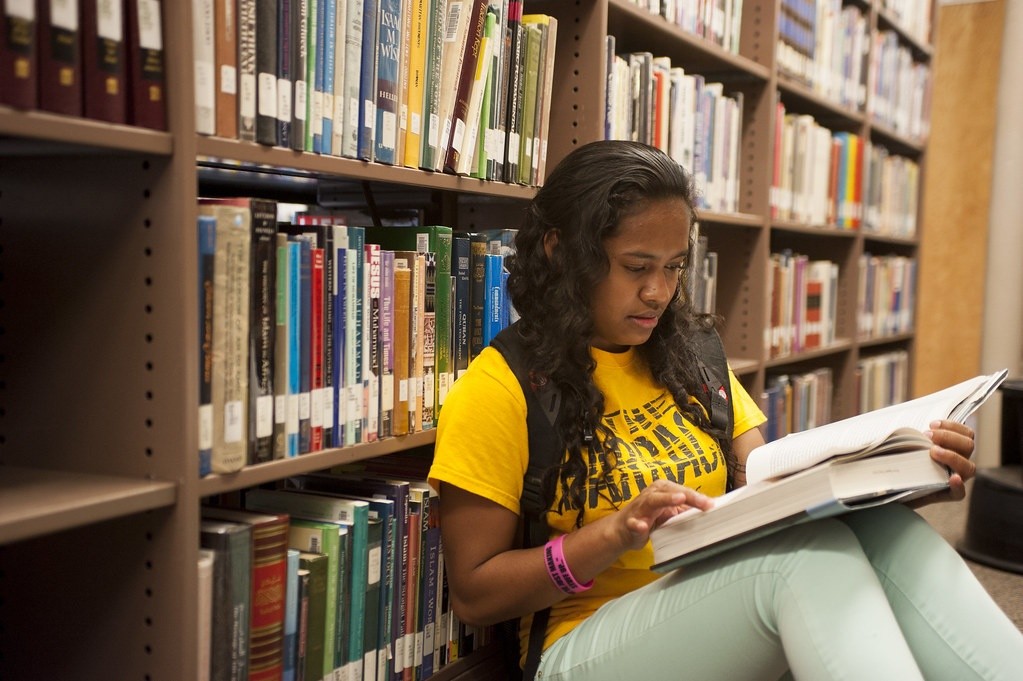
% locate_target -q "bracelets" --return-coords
[545,534,593,594]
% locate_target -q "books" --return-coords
[0,0,933,681]
[648,367,1008,572]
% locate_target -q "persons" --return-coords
[426,142,1022,681]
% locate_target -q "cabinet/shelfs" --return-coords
[0,2,939,681]
